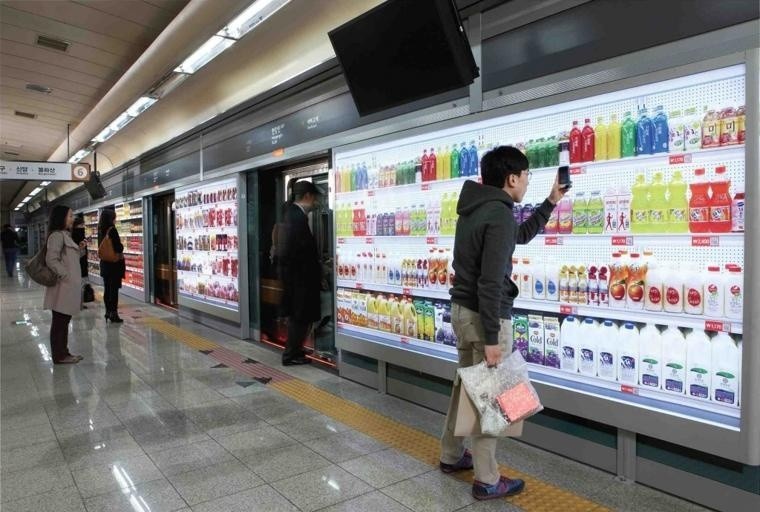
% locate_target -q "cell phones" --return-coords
[558,166,569,190]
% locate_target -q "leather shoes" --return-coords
[282,345,313,366]
[54,352,83,364]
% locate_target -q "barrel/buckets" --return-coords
[559,316,743,408]
[367,292,418,339]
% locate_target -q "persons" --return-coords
[277,180,328,366]
[270,194,313,346]
[318,249,334,291]
[41,204,90,363]
[1,224,19,277]
[70,217,95,302]
[436,145,573,501]
[97,208,127,323]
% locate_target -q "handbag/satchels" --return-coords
[445,355,544,440]
[96,235,123,263]
[24,245,60,287]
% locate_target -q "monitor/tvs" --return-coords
[84,171,107,200]
[326,0,480,126]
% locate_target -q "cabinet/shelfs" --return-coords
[174,173,240,310]
[83,198,144,290]
[331,62,745,419]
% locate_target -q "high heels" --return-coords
[105,312,123,323]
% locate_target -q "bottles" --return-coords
[337,92,745,409]
[175,184,239,303]
[83,200,145,289]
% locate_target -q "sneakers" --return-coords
[440,449,473,474]
[472,476,525,500]
